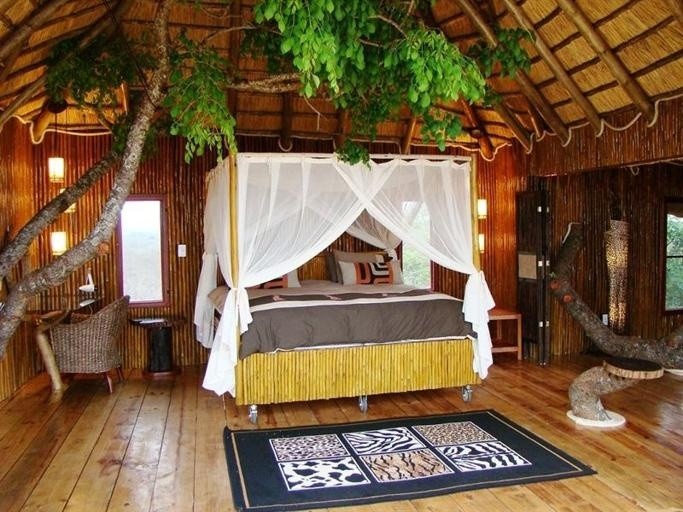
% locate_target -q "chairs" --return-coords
[47,295,130,394]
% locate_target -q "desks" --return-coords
[128,314,186,382]
[23,292,105,410]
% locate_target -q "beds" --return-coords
[209,150,482,426]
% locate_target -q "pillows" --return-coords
[247,250,404,291]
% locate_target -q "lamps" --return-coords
[44,98,67,184]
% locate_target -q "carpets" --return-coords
[223,407,598,511]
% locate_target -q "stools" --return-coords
[485,307,522,361]
